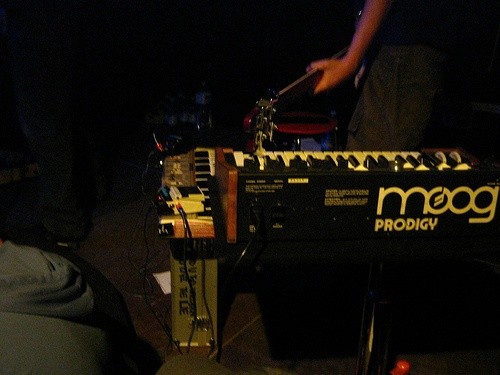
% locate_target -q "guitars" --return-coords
[243,43,352,153]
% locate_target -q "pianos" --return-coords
[156,143,500,261]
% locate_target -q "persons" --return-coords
[305,0,442,150]
[1,239,116,374]
[0,0,92,251]
